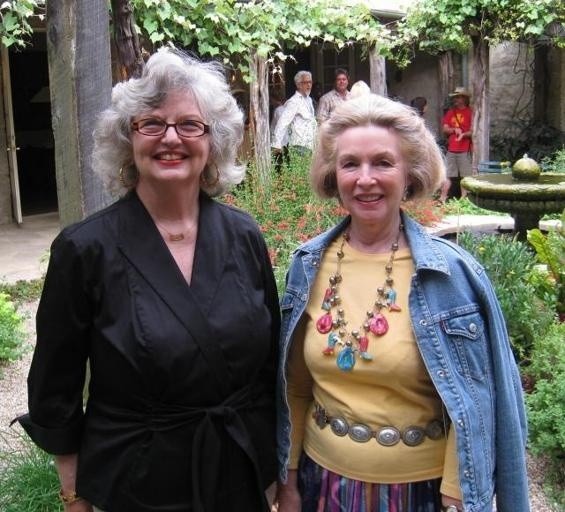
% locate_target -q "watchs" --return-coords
[441,504,459,512]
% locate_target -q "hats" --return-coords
[449,86,472,97]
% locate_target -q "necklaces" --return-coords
[316,224,405,371]
[157,221,196,242]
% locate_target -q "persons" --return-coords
[272,70,317,161]
[315,68,351,125]
[276,93,536,511]
[440,86,473,203]
[7,49,281,511]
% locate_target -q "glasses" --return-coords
[130,117,210,138]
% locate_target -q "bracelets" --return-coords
[58,488,81,504]
